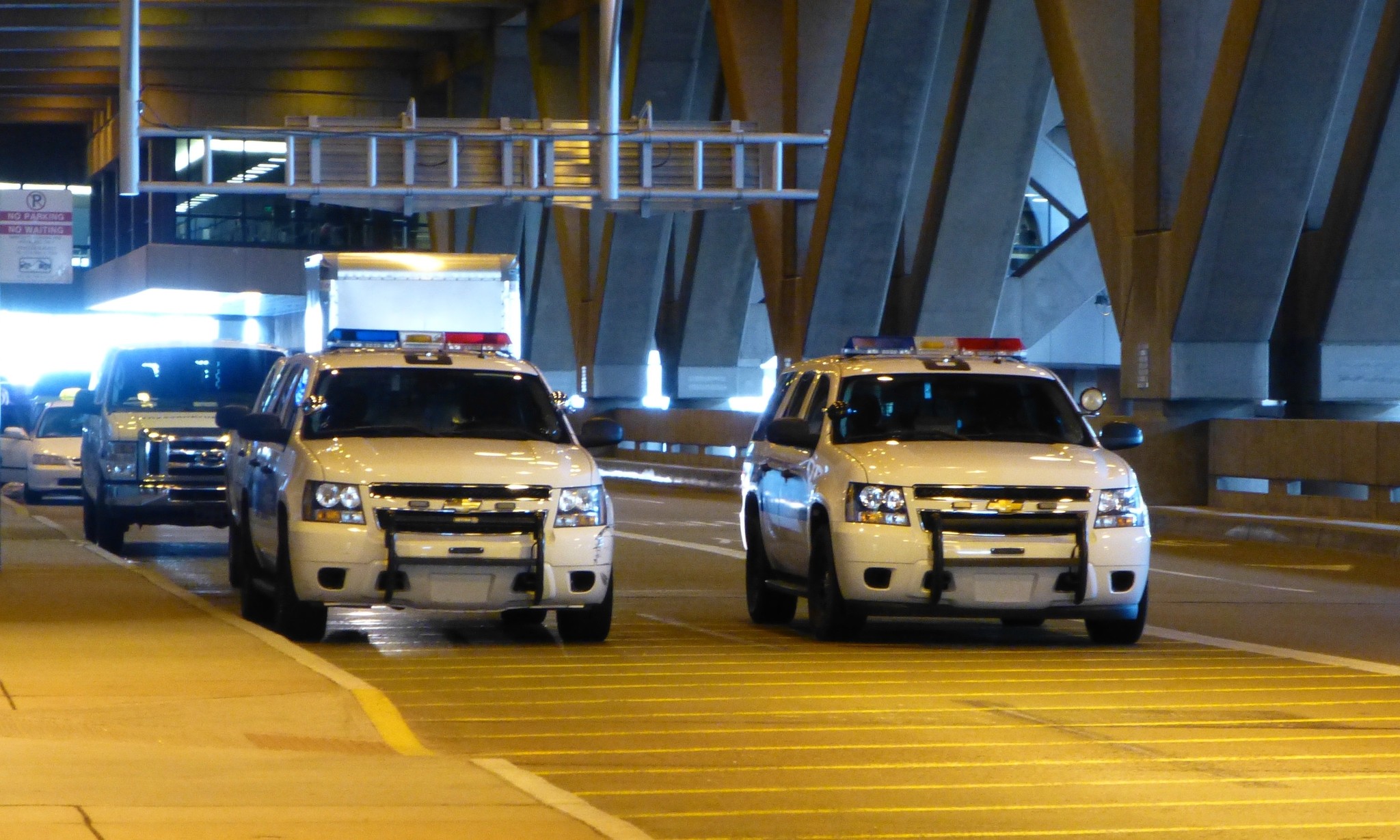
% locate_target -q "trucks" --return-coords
[302,250,523,362]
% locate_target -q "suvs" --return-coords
[739,333,1152,645]
[73,340,291,548]
[213,327,617,647]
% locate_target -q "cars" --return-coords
[1,373,83,505]
